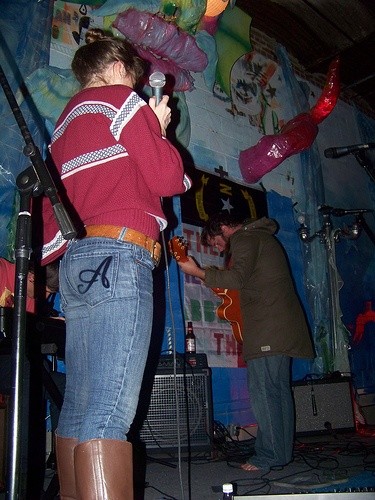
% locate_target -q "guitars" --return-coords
[168,235,243,345]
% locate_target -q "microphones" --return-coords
[149,72,166,107]
[325,143,375,159]
[332,208,369,217]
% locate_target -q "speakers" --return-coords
[128,352,214,455]
[290,377,355,436]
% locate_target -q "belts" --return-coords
[75,225,161,267]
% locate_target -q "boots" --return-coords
[55,428,133,500]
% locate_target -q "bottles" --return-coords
[185,322,196,354]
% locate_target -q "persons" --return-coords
[0,251,66,469]
[177,210,315,473]
[36,34,193,500]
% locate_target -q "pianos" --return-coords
[0,306,66,361]
